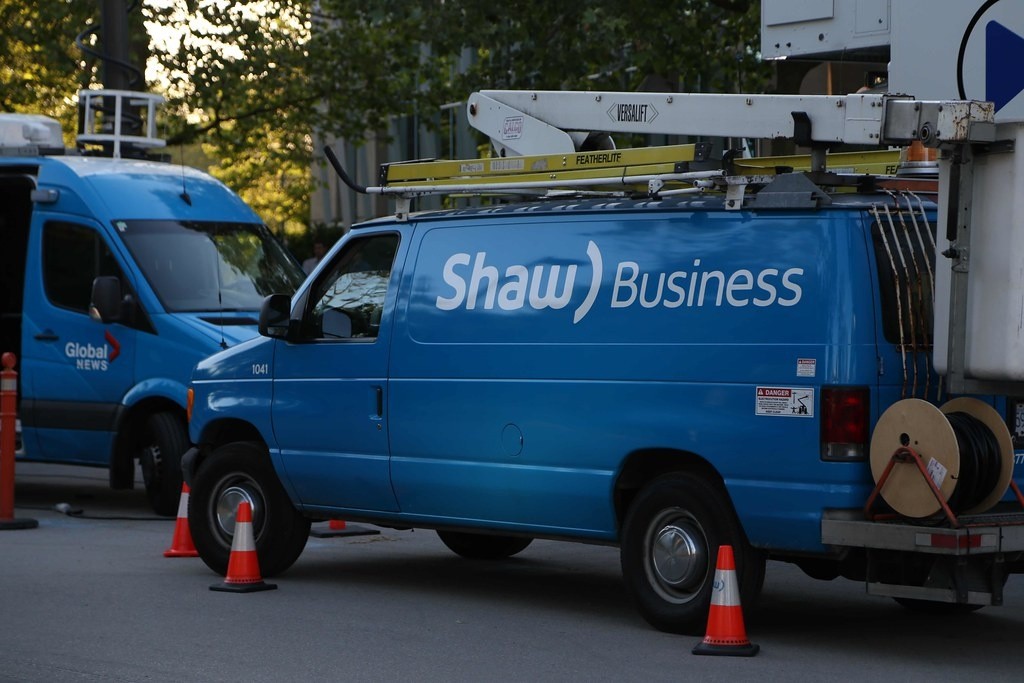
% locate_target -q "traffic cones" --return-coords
[690,543,760,658]
[311,517,382,537]
[163,482,200,556]
[209,500,278,594]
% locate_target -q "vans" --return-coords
[184,184,1024,633]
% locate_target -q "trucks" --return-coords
[0,86,312,522]
